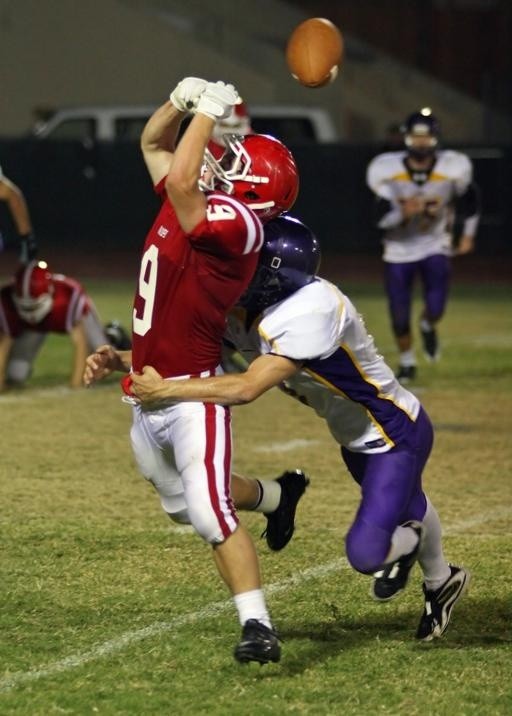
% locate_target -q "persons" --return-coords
[1,260,132,388]
[208,100,252,161]
[1,176,33,275]
[82,215,471,642]
[127,73,310,663]
[363,108,480,386]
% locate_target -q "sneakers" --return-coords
[397,365,416,384]
[104,322,131,350]
[415,563,470,642]
[259,469,309,551]
[418,320,437,358]
[234,619,284,663]
[370,519,427,602]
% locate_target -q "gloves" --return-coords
[195,80,242,122]
[170,76,208,114]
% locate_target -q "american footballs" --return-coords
[286,17,344,89]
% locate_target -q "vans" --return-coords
[36,100,332,146]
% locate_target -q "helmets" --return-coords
[13,260,54,323]
[234,214,321,308]
[197,133,300,221]
[399,111,441,162]
